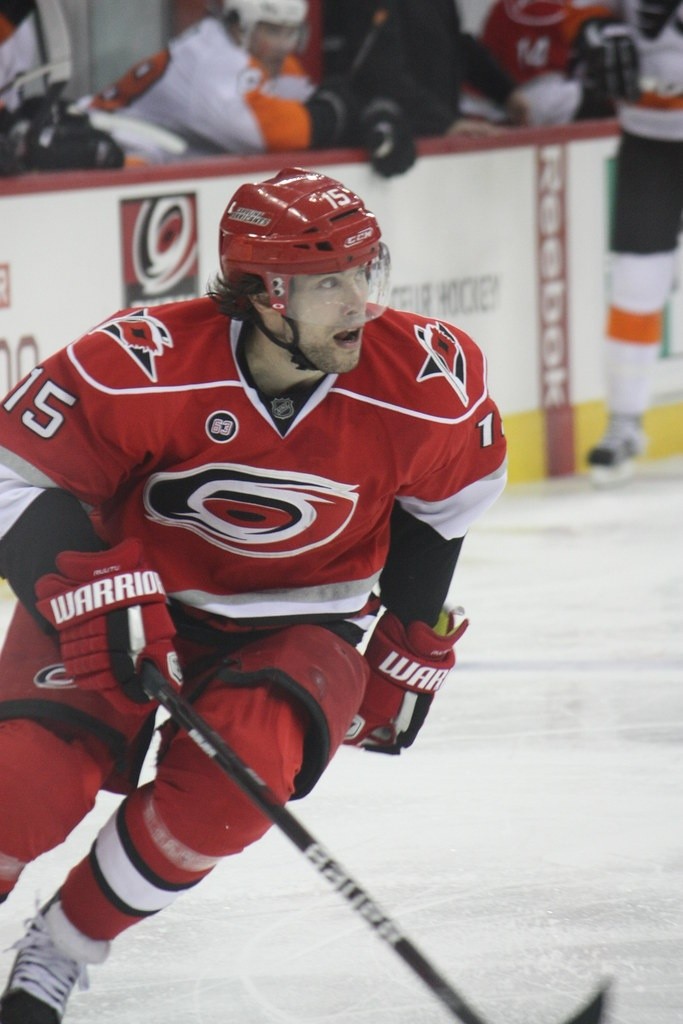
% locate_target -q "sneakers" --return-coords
[2,892,85,1024]
[587,419,644,485]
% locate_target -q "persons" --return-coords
[0,159,506,1024]
[368,3,533,137]
[574,0,683,484]
[67,0,360,153]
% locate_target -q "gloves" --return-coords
[565,17,638,108]
[337,608,469,756]
[306,78,359,147]
[358,101,417,177]
[32,537,182,716]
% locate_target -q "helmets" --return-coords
[218,167,380,317]
[220,1,312,55]
